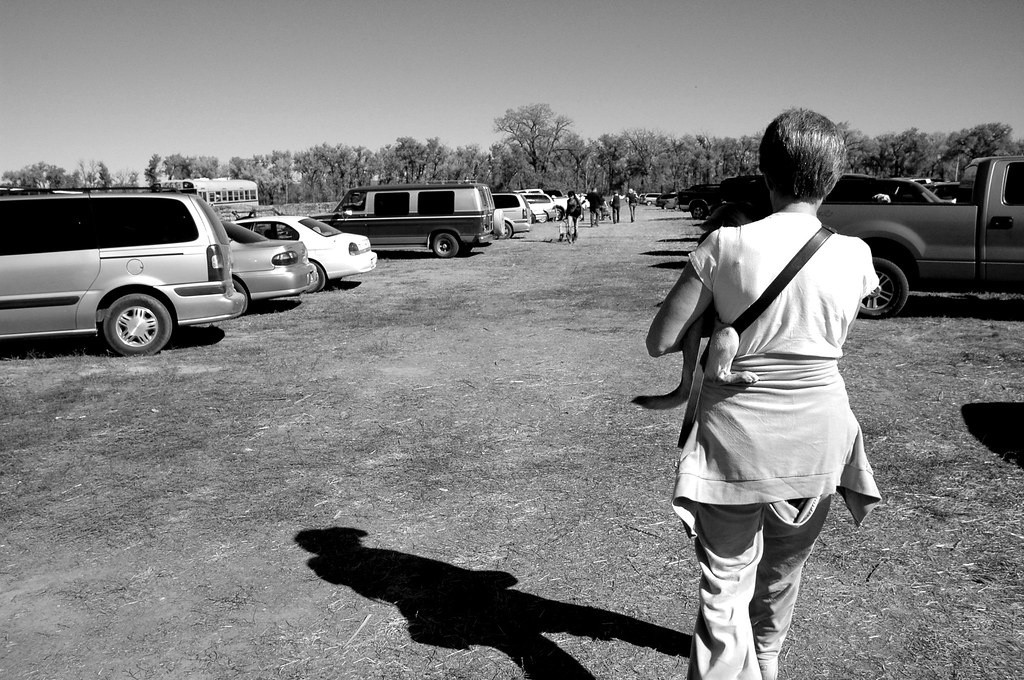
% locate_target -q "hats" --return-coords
[629,189,633,194]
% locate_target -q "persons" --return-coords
[646,108,882,680]
[559,187,639,244]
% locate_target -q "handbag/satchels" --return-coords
[572,205,581,218]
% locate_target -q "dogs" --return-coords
[627,204,760,411]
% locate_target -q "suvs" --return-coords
[0,182,247,356]
[308,180,506,259]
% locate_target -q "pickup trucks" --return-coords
[816,155,1024,321]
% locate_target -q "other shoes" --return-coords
[594,222,598,226]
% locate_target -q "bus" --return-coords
[157,177,259,218]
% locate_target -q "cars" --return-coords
[491,189,590,240]
[210,202,315,315]
[638,173,967,236]
[228,216,378,293]
